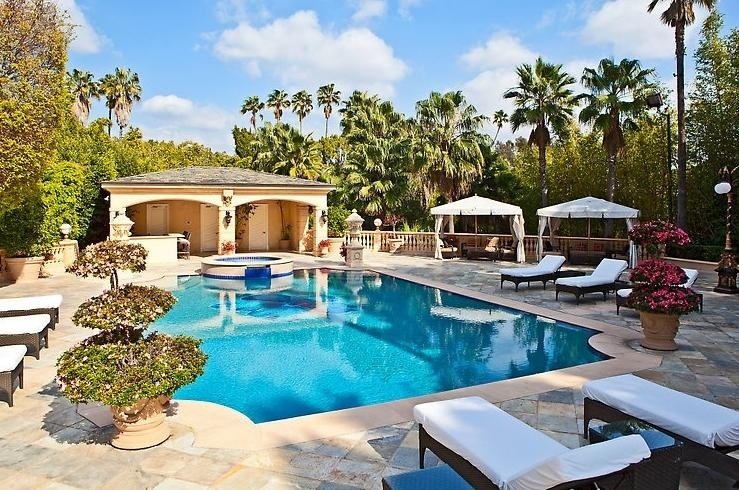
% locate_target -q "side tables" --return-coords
[590,418,682,490]
[383,462,475,489]
[554,270,586,284]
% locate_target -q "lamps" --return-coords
[224,294,232,310]
[319,288,327,303]
[59,221,73,239]
[226,210,232,226]
[374,218,383,231]
[321,210,328,225]
[374,275,382,287]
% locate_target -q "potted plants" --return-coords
[235,202,257,253]
[221,240,235,255]
[55,240,209,450]
[320,239,330,254]
[279,224,293,250]
[0,182,51,283]
[384,213,405,252]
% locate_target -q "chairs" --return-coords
[461,235,501,255]
[414,396,653,490]
[0,314,51,360]
[581,373,739,490]
[499,239,518,261]
[544,241,553,251]
[499,255,566,292]
[1,295,62,330]
[555,258,628,306]
[616,268,698,314]
[606,244,629,258]
[132,230,191,259]
[439,238,458,259]
[0,345,28,407]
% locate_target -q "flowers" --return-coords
[629,257,701,314]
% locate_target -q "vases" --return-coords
[47,253,53,260]
[638,310,681,350]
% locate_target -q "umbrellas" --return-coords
[430,193,522,248]
[536,196,639,256]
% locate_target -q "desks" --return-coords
[464,246,498,263]
